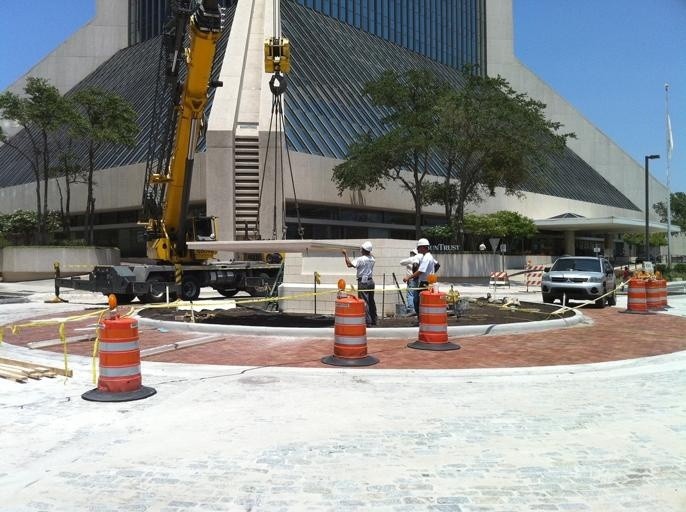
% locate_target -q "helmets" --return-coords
[417,238,430,246]
[362,242,373,252]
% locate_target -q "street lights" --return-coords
[644,154,659,259]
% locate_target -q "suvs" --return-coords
[540,256,616,308]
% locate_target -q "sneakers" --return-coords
[407,311,416,316]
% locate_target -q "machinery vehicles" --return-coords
[53,0,303,302]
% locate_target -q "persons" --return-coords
[399,251,424,317]
[342,242,379,327]
[402,238,435,323]
[623,266,631,280]
